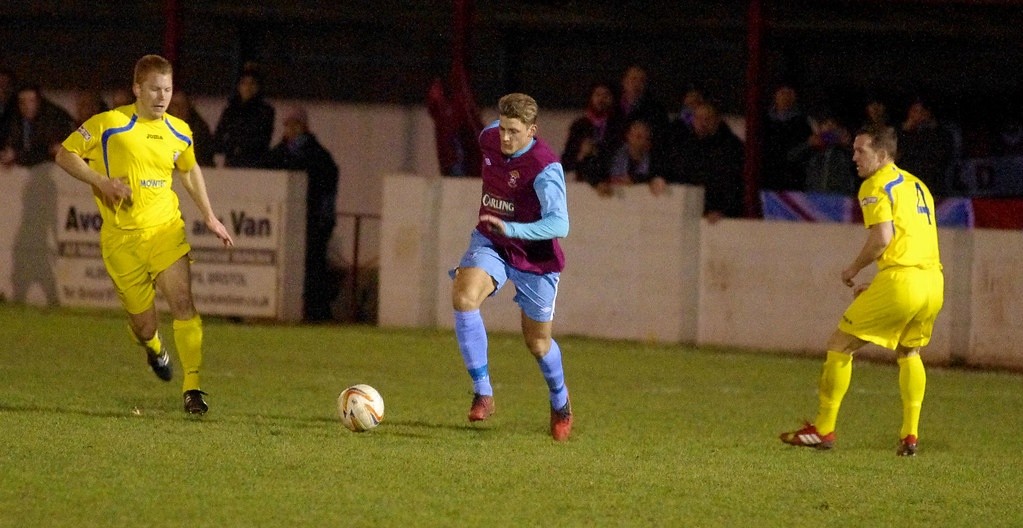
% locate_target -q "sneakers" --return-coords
[779,421,835,450]
[467,392,495,420]
[550,392,572,443]
[182,389,209,417]
[896,435,917,455]
[146,338,172,380]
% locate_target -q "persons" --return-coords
[56,54,234,416]
[449,93,572,441]
[779,124,945,456]
[430,79,484,176]
[761,83,958,198]
[168,64,339,323]
[0,67,135,167]
[561,64,746,222]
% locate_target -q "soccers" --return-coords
[338,383,385,433]
